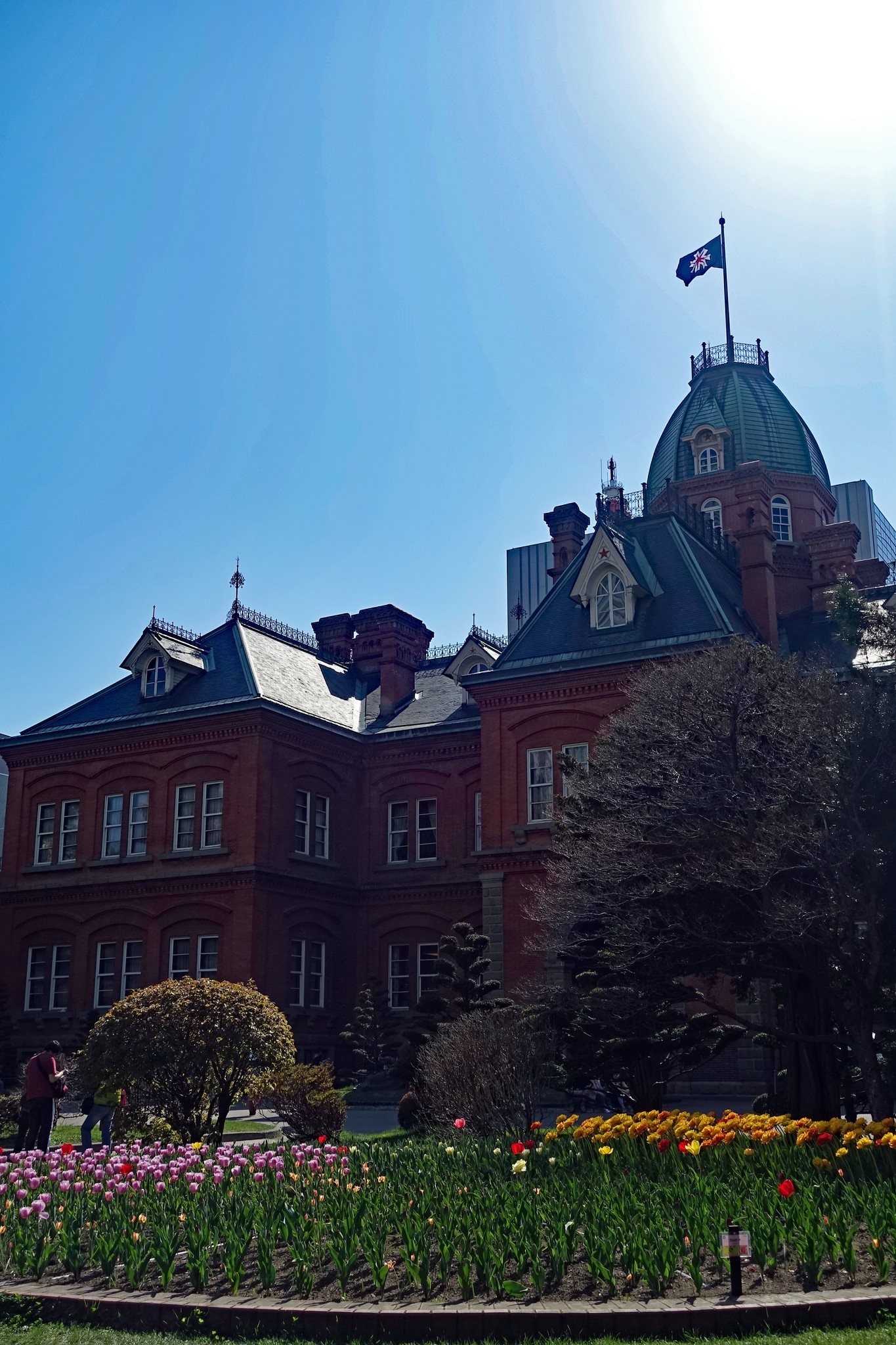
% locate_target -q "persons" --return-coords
[81,1066,122,1157]
[398,1080,437,1131]
[843,1062,896,1128]
[565,1067,634,1114]
[13,1041,69,1155]
[246,1060,265,1116]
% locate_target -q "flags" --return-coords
[676,234,724,287]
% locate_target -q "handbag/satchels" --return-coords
[43,1071,66,1099]
[80,1096,94,1115]
